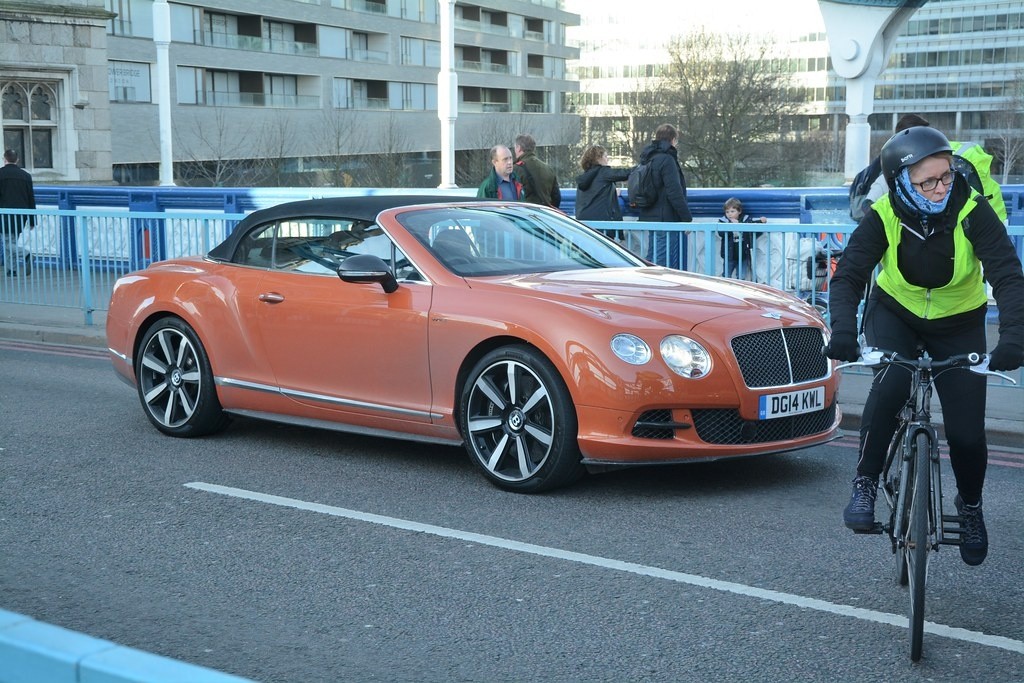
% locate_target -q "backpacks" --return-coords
[628,152,665,208]
[849,156,880,225]
[948,141,1008,224]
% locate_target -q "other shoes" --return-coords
[7,270,16,276]
[26,254,34,276]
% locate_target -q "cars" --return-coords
[105,183,847,494]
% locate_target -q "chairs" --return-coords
[432,229,474,265]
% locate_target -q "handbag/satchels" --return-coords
[17,220,39,251]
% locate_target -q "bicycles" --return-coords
[820,344,1018,662]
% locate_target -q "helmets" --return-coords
[881,126,955,192]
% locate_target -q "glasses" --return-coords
[911,168,956,191]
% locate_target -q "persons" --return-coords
[0,149,38,278]
[334,168,353,187]
[477,133,562,209]
[638,123,692,268]
[718,197,767,280]
[827,113,1024,565]
[575,145,637,241]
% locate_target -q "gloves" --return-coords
[826,331,861,362]
[989,344,1024,371]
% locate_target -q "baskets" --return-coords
[786,257,826,291]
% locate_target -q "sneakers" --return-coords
[844,468,879,523]
[954,493,988,566]
